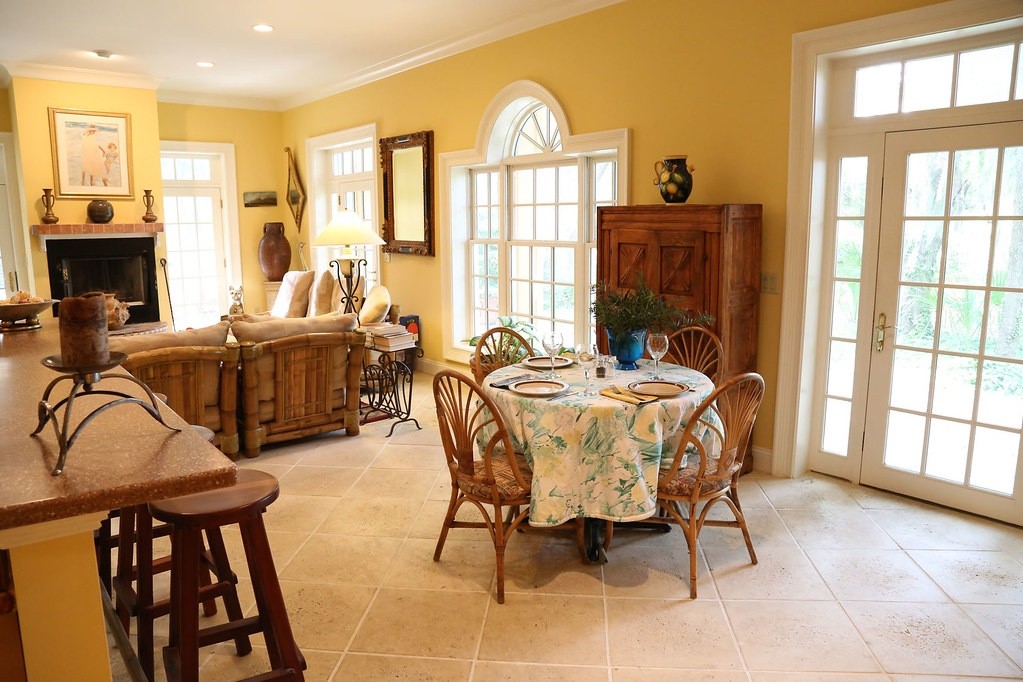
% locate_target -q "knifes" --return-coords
[512,365,543,373]
[546,391,579,401]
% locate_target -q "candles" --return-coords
[57,292,111,366]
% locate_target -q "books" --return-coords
[360,322,416,351]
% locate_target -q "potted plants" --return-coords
[584,271,715,371]
[459,317,541,387]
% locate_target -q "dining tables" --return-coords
[476,353,725,566]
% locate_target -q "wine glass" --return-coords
[575,343,599,398]
[541,332,564,379]
[646,333,669,381]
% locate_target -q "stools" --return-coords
[112,504,255,682]
[148,471,307,682]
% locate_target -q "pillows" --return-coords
[270,270,314,319]
[358,283,391,324]
[306,271,332,318]
[330,275,364,315]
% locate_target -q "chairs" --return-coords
[602,372,765,600]
[475,327,537,386]
[432,369,590,604]
[650,326,724,394]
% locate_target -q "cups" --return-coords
[596,354,617,379]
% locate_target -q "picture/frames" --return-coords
[47,106,136,201]
[284,147,304,233]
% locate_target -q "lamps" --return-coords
[97,51,111,58]
[311,205,387,327]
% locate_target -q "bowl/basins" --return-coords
[0,299,61,320]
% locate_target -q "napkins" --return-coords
[489,374,546,390]
[599,386,659,406]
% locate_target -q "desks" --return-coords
[358,343,425,438]
[0,317,237,682]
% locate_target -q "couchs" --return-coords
[108,318,241,462]
[258,269,400,394]
[219,310,368,459]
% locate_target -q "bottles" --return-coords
[88,199,114,224]
[257,222,292,282]
[657,155,694,206]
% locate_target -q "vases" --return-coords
[41,188,59,224]
[142,190,157,223]
[257,222,292,282]
[104,294,130,330]
[653,154,696,205]
[86,200,114,224]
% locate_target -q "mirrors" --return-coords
[379,130,436,256]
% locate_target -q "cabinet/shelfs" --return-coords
[594,204,763,475]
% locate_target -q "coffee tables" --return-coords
[107,322,169,337]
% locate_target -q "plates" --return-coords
[522,355,574,368]
[508,379,570,397]
[628,379,689,397]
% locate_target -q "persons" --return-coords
[81,124,106,186]
[102,143,118,186]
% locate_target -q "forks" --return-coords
[609,385,646,401]
